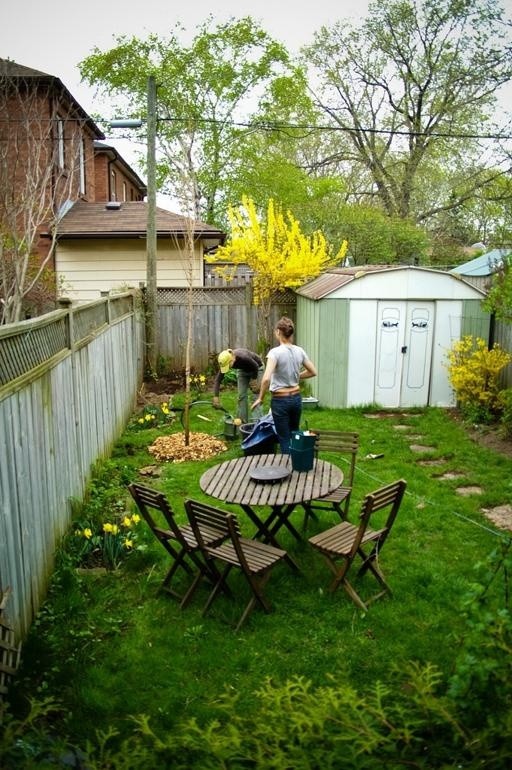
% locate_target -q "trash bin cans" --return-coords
[239,423,277,457]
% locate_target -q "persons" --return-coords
[211,347,265,425]
[250,317,318,453]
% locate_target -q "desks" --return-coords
[199,453,345,577]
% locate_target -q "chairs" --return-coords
[308,478,408,613]
[303,430,359,533]
[185,499,288,635]
[128,484,229,611]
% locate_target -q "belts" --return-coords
[272,389,300,397]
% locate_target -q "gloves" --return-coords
[250,379,260,393]
[251,399,263,412]
[213,397,221,408]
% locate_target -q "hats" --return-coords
[218,350,233,374]
